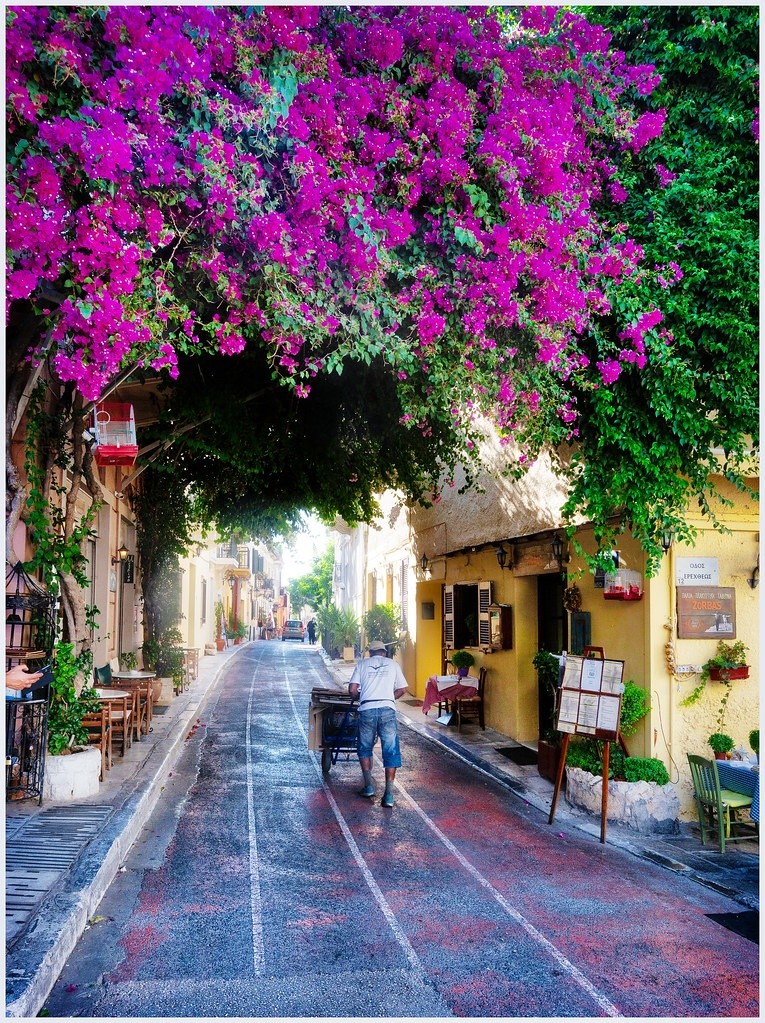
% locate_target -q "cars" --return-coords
[281,620,306,643]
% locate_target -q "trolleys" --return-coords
[319,692,379,772]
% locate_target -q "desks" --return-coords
[693,754,759,845]
[109,672,156,734]
[422,675,478,714]
[76,688,130,757]
[170,647,197,681]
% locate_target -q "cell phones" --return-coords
[23,665,54,693]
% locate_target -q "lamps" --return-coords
[111,543,129,566]
[192,544,202,557]
[421,552,432,572]
[659,527,675,554]
[497,546,514,571]
[549,534,569,567]
[224,569,236,588]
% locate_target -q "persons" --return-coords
[6,666,44,691]
[307,617,317,644]
[713,611,731,631]
[349,641,409,806]
[267,617,272,640]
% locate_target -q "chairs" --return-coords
[170,646,201,695]
[69,669,156,782]
[438,659,488,732]
[687,751,758,853]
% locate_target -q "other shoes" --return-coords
[381,793,394,807]
[359,786,375,796]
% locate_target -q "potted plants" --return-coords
[337,605,361,662]
[534,649,571,781]
[234,619,246,644]
[214,602,225,652]
[27,643,103,802]
[749,729,759,765]
[145,641,174,706]
[679,639,750,731]
[452,651,476,677]
[226,631,238,646]
[708,733,734,760]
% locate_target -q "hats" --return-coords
[368,641,388,652]
